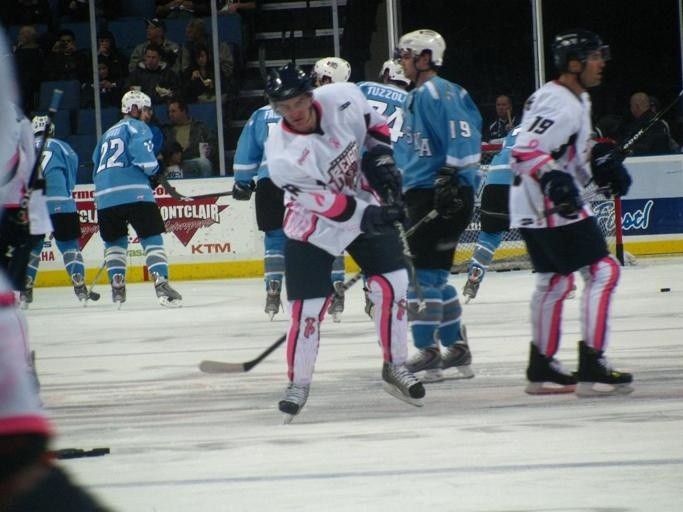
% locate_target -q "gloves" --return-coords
[232,180,257,200]
[360,204,406,233]
[435,167,464,216]
[360,145,403,202]
[540,169,582,220]
[590,142,633,200]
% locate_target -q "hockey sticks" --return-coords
[199,209,438,373]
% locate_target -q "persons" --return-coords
[232,28,636,425]
[1,1,257,510]
[609,93,681,155]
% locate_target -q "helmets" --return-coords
[264,55,354,115]
[555,32,603,66]
[122,87,151,117]
[29,115,56,136]
[380,29,445,86]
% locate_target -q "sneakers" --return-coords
[19,287,32,302]
[277,384,310,414]
[74,285,88,299]
[112,286,126,302]
[155,282,182,300]
[464,281,478,298]
[576,357,631,384]
[380,341,466,397]
[527,354,578,385]
[328,295,344,313]
[265,294,279,313]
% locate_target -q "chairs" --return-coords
[0,0,252,186]
